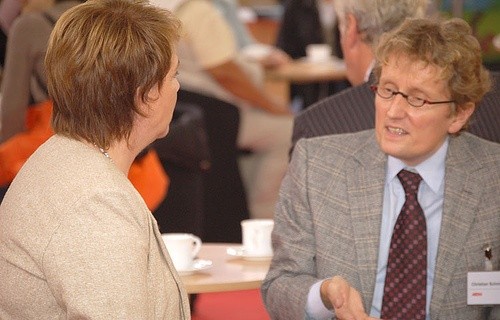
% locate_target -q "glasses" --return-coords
[369,80,456,107]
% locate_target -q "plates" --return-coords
[226,247,274,261]
[176,259,213,275]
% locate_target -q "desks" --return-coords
[178,243,272,319]
[268,57,345,103]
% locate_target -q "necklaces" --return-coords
[89,140,113,162]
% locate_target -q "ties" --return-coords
[380,169,427,320]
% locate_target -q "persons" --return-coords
[0,0,354,242]
[0,0,191,320]
[290,0,500,161]
[261,18,500,320]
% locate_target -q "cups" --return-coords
[162,233,202,267]
[307,44,331,69]
[241,219,274,253]
